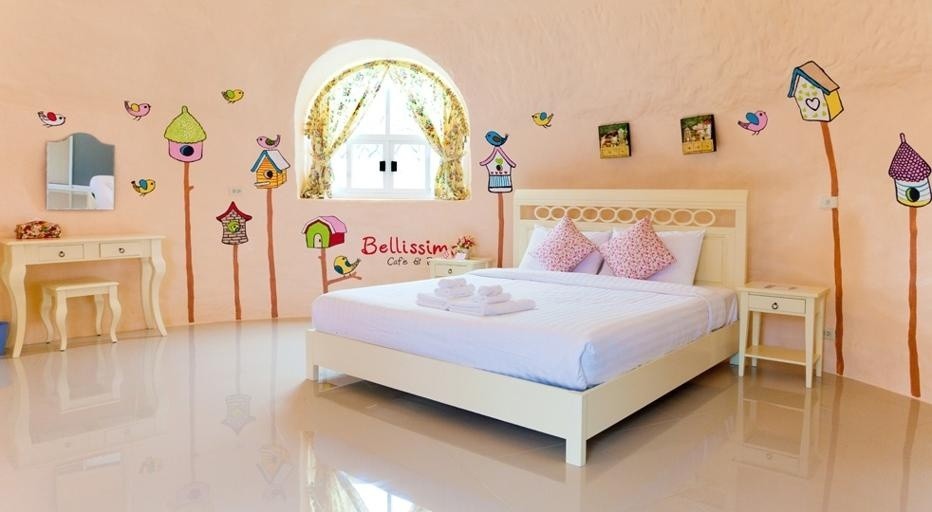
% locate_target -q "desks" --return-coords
[5,238,176,359]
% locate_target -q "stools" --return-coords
[43,273,124,349]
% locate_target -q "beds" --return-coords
[305,186,751,467]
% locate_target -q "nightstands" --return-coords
[739,279,827,385]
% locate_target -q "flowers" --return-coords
[452,235,475,259]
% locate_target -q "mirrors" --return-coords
[43,132,122,215]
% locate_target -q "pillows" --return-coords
[598,230,705,284]
[518,224,609,277]
[538,214,596,273]
[597,216,675,280]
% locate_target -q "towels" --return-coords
[418,280,536,317]
